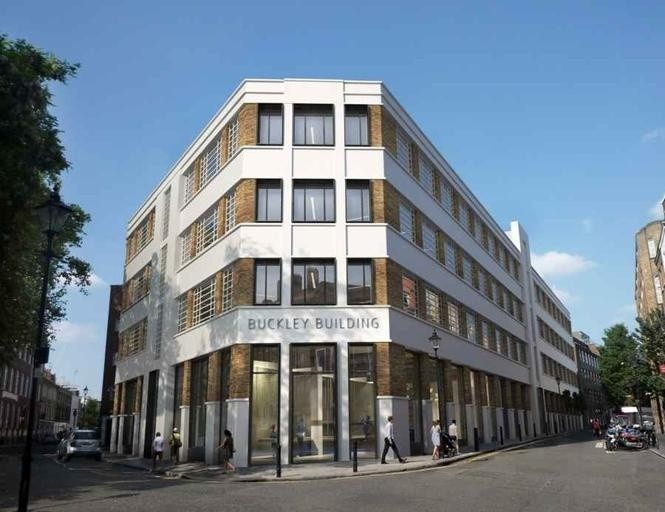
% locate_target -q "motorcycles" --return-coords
[606,423,650,453]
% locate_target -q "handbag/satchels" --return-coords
[174,438,182,448]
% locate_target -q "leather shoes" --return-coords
[399,458,408,463]
[381,461,389,464]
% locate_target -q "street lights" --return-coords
[428,326,449,433]
[556,374,564,435]
[17,182,74,512]
[82,385,88,429]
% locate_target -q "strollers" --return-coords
[440,431,458,458]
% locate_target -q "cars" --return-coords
[56,429,103,462]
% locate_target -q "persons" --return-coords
[380,415,408,463]
[296,417,305,450]
[270,423,277,460]
[430,419,442,460]
[360,415,372,442]
[593,417,602,439]
[169,427,182,464]
[449,419,462,455]
[151,431,164,468]
[215,429,236,473]
